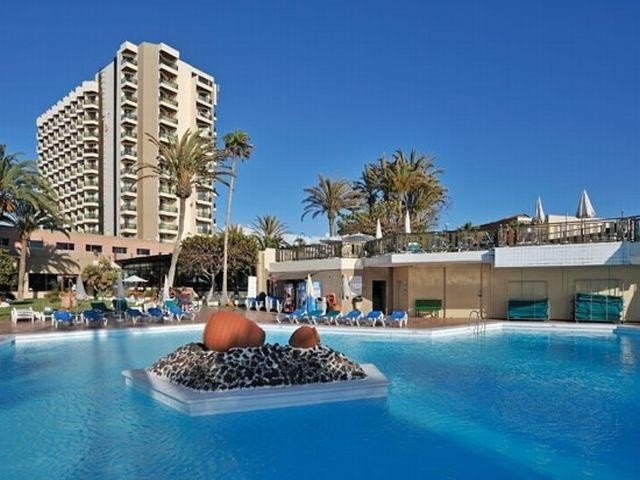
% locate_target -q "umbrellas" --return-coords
[122,274,149,290]
[376,218,383,239]
[117,273,125,322]
[576,189,596,218]
[405,211,411,233]
[74,274,87,313]
[535,196,546,223]
[304,274,314,315]
[163,274,170,301]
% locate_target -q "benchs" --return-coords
[413,300,442,318]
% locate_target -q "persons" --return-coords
[168,286,192,312]
[285,293,294,314]
[325,292,342,320]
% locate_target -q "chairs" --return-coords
[276,308,409,329]
[10,299,196,328]
